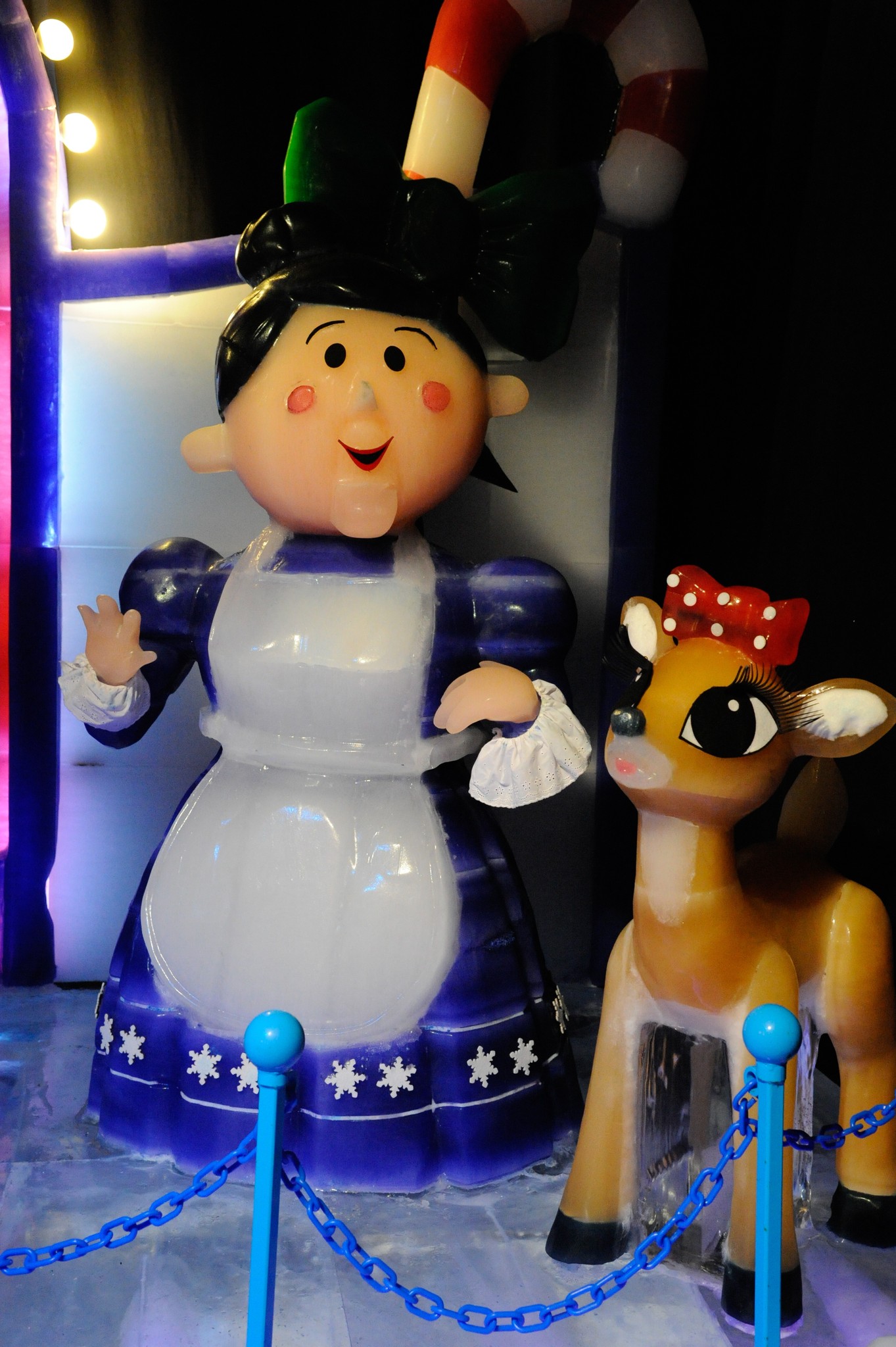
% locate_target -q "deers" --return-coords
[544,561,895,1330]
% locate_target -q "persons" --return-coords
[76,157,593,1196]
